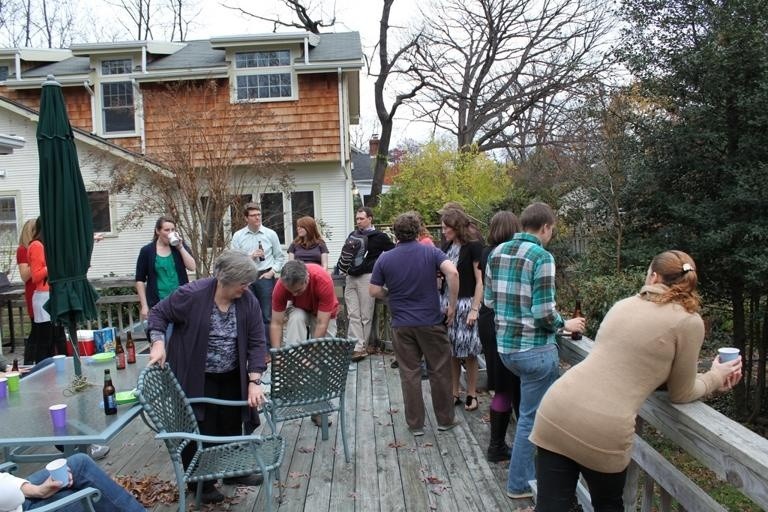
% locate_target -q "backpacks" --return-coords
[337,228,378,277]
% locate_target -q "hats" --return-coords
[435,201,464,216]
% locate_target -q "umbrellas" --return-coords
[35,73,102,381]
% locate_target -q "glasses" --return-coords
[248,214,261,217]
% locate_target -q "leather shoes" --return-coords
[391,361,398,368]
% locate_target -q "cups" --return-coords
[716,348,738,363]
[48,404,67,429]
[45,458,70,488]
[0,377,7,399]
[166,232,179,247]
[5,371,19,393]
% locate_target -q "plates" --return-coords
[90,352,115,363]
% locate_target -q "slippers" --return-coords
[465,395,478,411]
[453,395,462,406]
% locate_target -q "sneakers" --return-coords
[408,426,424,436]
[351,350,368,361]
[86,444,111,461]
[188,484,224,505]
[506,490,533,498]
[311,414,331,427]
[437,416,460,431]
[223,474,263,486]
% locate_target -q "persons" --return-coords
[228,202,286,364]
[483,201,587,499]
[143,251,269,504]
[28,214,56,363]
[270,260,339,426]
[521,249,745,512]
[1,452,148,511]
[16,218,69,365]
[134,216,197,346]
[286,216,330,275]
[333,201,521,464]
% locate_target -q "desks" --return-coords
[0,352,172,466]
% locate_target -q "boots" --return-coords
[487,406,512,462]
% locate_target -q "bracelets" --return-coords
[249,378,261,385]
[177,246,183,250]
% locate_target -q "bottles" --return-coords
[10,360,18,371]
[113,335,125,370]
[101,369,117,415]
[572,298,583,341]
[258,241,264,261]
[125,331,135,364]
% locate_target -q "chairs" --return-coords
[1,457,113,512]
[134,361,288,511]
[260,338,357,463]
